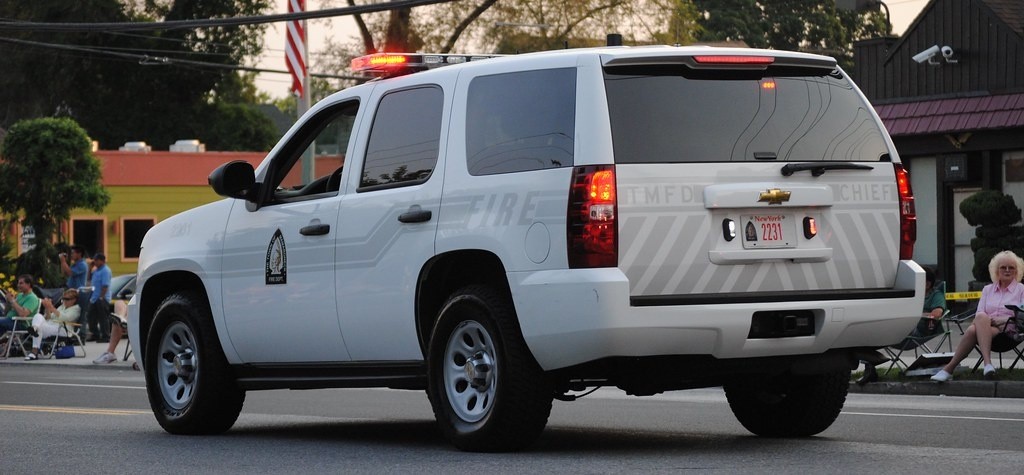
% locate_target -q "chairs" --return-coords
[945,304,1024,374]
[882,277,952,376]
[0,286,136,361]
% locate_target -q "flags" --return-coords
[284,0,305,100]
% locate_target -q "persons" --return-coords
[24,288,81,360]
[92,295,127,364]
[931,250,1024,382]
[0,274,39,337]
[851,267,945,384]
[85,253,112,343]
[59,245,88,346]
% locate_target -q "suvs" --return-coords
[124,32,926,446]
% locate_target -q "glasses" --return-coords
[61,297,75,300]
[998,265,1016,270]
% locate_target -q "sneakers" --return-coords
[96,336,110,343]
[86,334,100,340]
[856,366,880,385]
[983,364,995,377]
[931,369,954,382]
[93,351,117,364]
[133,362,139,370]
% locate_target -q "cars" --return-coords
[87,272,137,340]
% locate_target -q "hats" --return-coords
[93,253,106,260]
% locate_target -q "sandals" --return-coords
[27,325,39,337]
[24,352,38,360]
